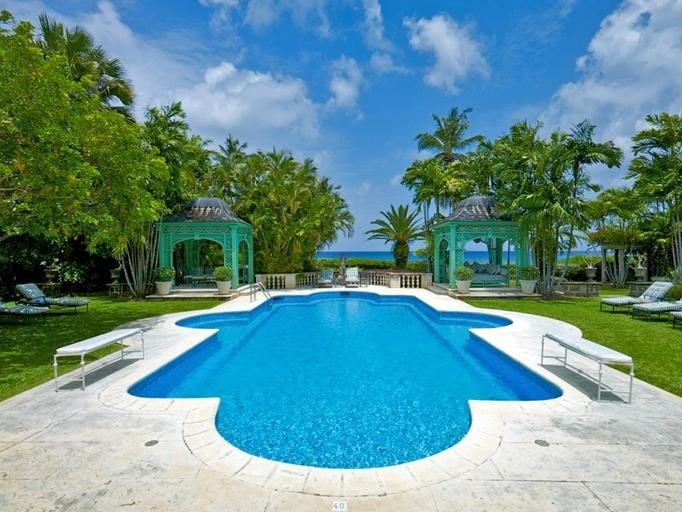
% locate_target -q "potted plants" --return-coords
[38,254,59,286]
[583,255,601,282]
[152,264,176,295]
[213,266,232,294]
[625,251,647,281]
[519,266,540,294]
[452,267,475,294]
[508,265,517,287]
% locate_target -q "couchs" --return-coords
[463,261,508,288]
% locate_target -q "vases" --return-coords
[110,269,121,284]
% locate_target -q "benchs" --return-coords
[541,333,635,405]
[53,328,144,392]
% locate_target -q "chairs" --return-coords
[670,310,682,331]
[15,282,90,315]
[183,266,217,288]
[344,267,360,288]
[632,297,682,321]
[600,281,674,314]
[314,269,336,288]
[0,297,50,327]
